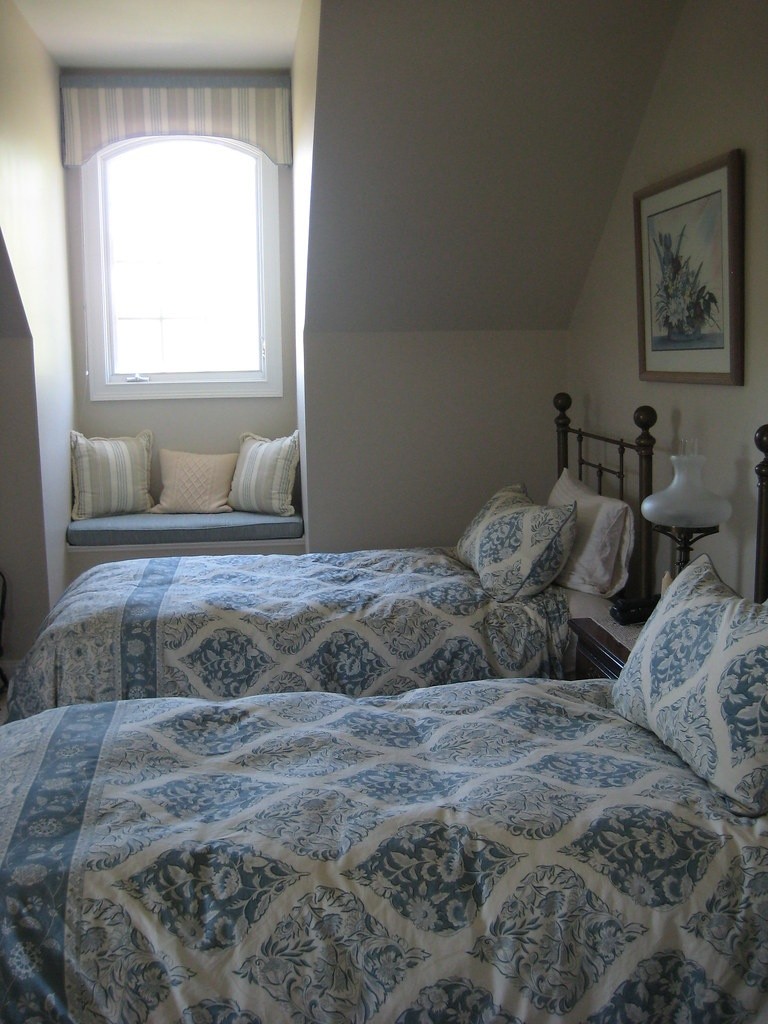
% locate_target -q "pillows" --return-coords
[608,553,768,818]
[452,482,580,603]
[69,428,154,521]
[547,468,635,597]
[227,430,300,516]
[150,448,238,514]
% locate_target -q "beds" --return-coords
[0,392,658,726]
[0,425,768,1024]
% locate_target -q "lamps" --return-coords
[640,438,732,577]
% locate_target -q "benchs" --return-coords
[66,510,304,548]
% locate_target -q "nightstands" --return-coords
[567,615,647,679]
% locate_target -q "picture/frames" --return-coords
[632,148,747,388]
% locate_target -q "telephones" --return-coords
[609,592,662,626]
